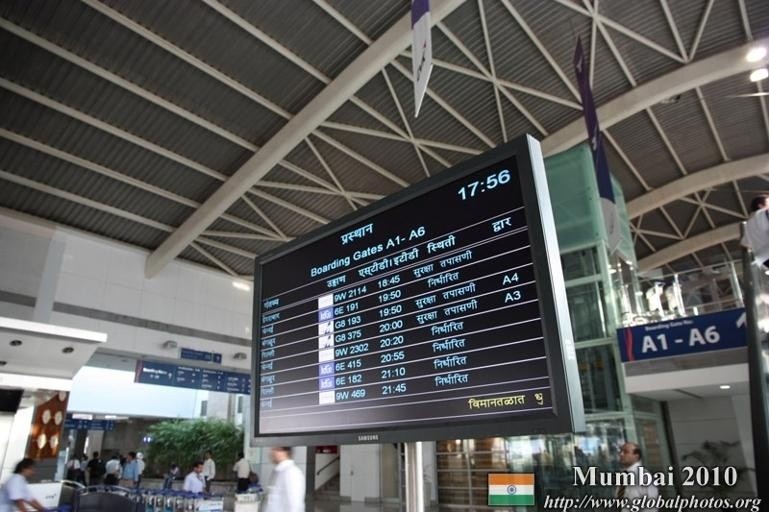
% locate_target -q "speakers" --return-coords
[0,388,24,413]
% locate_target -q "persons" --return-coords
[66,443,305,511]
[665,277,683,316]
[740,195,769,271]
[560,436,572,475]
[540,449,553,476]
[615,442,682,512]
[644,280,664,317]
[0,458,45,512]
[577,445,590,469]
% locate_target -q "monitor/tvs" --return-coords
[250,134,586,444]
[134,360,251,394]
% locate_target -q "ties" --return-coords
[617,472,626,511]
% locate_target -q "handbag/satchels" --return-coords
[68,460,74,471]
[113,464,121,479]
